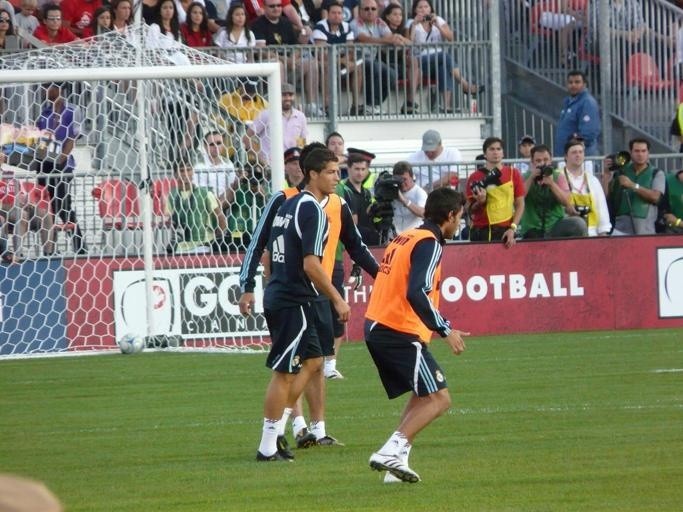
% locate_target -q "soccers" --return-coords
[120,332,144,353]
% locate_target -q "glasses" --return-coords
[364,7,376,11]
[269,4,281,8]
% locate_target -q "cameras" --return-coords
[605,150,632,171]
[423,14,433,22]
[536,164,554,178]
[576,205,592,214]
[469,167,502,192]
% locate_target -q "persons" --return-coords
[256,147,350,462]
[239,141,379,451]
[323,240,364,380]
[362,188,464,485]
[0,1,683,258]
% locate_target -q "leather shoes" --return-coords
[463,84,483,95]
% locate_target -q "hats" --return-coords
[281,83,295,94]
[347,148,375,162]
[520,135,536,144]
[284,148,302,163]
[422,131,440,151]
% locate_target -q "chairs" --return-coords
[340,46,439,90]
[0,175,178,258]
[530,0,683,109]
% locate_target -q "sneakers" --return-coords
[306,104,329,117]
[257,429,336,460]
[351,104,417,115]
[324,369,343,380]
[369,453,420,483]
